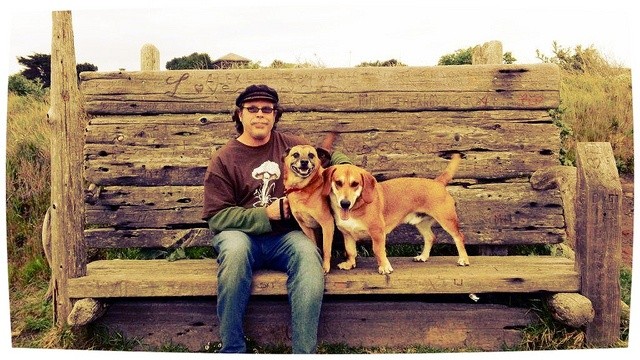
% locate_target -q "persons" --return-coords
[201,84,326,354]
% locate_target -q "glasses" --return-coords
[240,106,278,114]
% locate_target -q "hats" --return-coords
[235,85,278,104]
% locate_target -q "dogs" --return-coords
[321,153,470,274]
[280,144,335,276]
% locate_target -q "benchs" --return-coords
[42,11,621,349]
